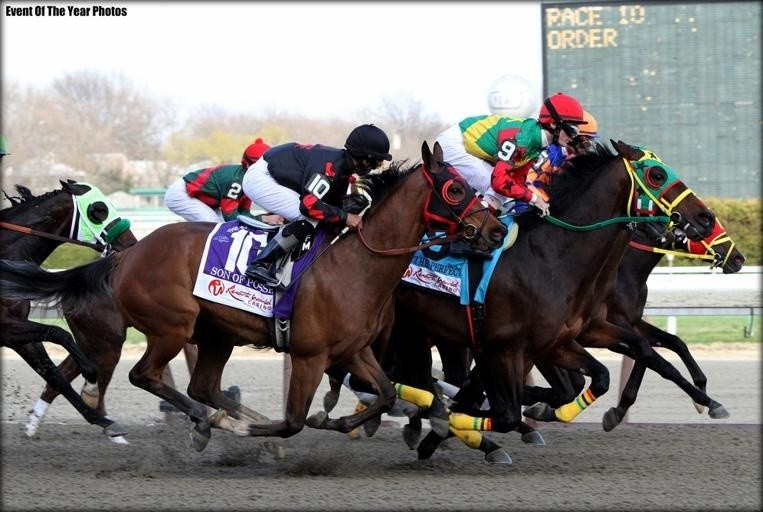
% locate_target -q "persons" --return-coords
[502,109,600,213]
[163,138,286,226]
[241,124,393,292]
[433,92,588,259]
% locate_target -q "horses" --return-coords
[0,135,748,467]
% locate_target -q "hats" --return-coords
[538,91,599,139]
[241,138,271,167]
[344,124,393,162]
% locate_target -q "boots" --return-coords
[449,241,493,263]
[244,226,299,290]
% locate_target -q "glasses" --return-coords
[562,121,593,150]
